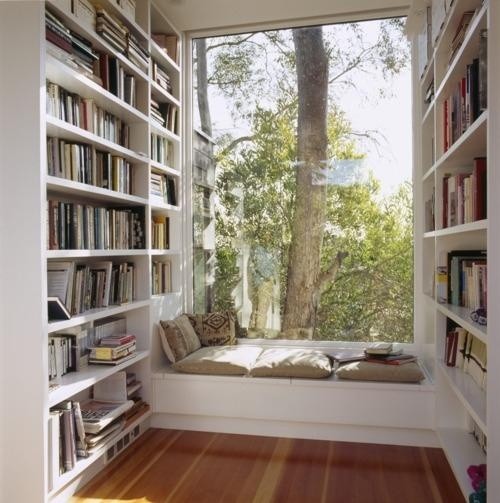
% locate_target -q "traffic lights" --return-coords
[335,362,425,382]
[185,310,239,345]
[157,314,201,364]
[170,346,265,376]
[253,350,334,378]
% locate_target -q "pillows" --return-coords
[335,362,425,382]
[157,314,201,364]
[185,310,239,345]
[253,350,334,378]
[170,346,265,376]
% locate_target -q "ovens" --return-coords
[150,351,435,447]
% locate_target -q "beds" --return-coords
[150,351,435,447]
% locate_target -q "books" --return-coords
[421,0,488,396]
[47,261,136,321]
[364,341,417,365]
[47,199,146,250]
[152,216,170,249]
[151,57,173,94]
[47,137,136,197]
[49,372,148,491]
[46,0,151,75]
[150,98,171,129]
[151,133,174,167]
[46,82,130,153]
[46,12,137,113]
[48,316,138,386]
[151,171,176,206]
[152,261,173,295]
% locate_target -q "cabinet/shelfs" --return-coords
[403,2,500,503]
[1,1,187,503]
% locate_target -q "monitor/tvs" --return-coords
[421,0,488,396]
[48,316,150,476]
[364,341,417,365]
